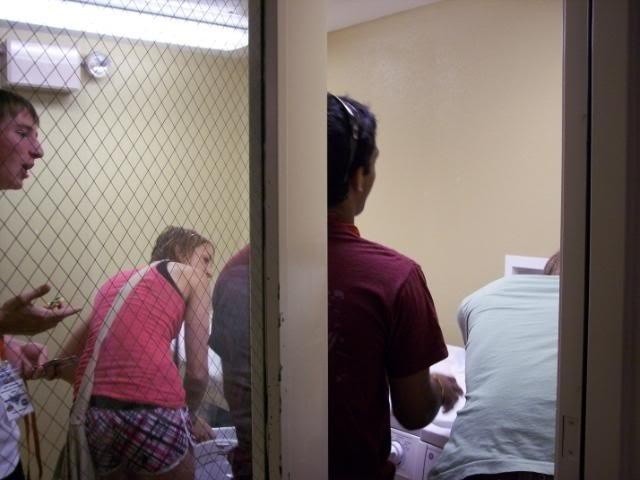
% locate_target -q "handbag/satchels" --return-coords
[53,415,95,480]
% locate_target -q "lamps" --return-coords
[82,50,111,78]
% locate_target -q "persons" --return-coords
[0,88,86,480]
[205,93,464,480]
[423,250,561,480]
[58,225,217,480]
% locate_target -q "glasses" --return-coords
[328,93,359,180]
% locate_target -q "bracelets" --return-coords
[434,375,445,404]
